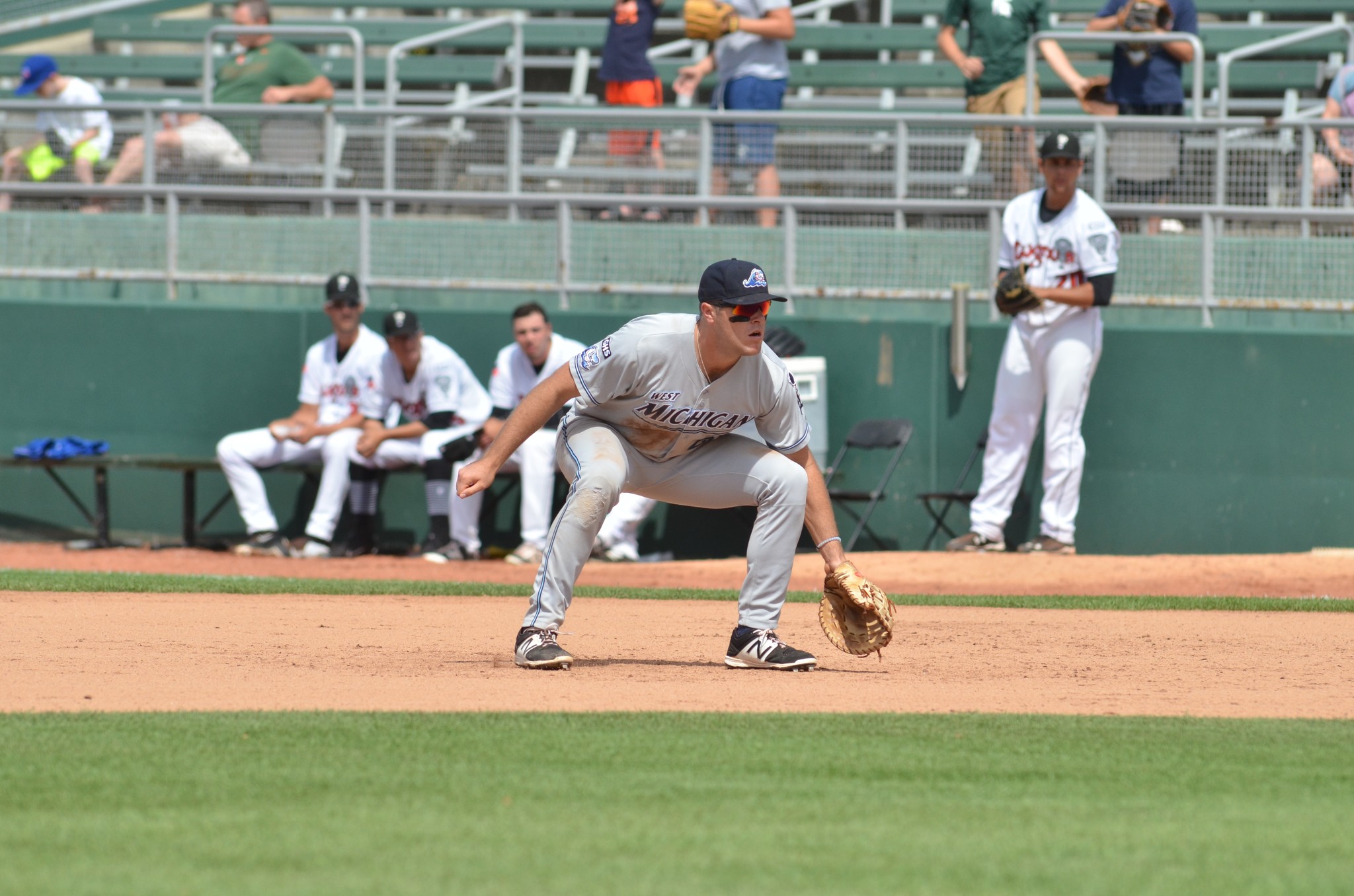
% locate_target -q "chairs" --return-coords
[824,416,913,551]
[917,427,1019,553]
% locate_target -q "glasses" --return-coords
[705,300,772,318]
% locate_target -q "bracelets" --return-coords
[813,537,844,551]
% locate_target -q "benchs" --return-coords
[0,0,1354,228]
[0,449,320,550]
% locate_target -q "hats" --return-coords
[698,258,787,305]
[385,311,416,337]
[15,55,56,96]
[1041,131,1079,159]
[327,274,359,301]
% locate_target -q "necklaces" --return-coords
[696,325,723,384]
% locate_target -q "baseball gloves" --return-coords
[1117,0,1175,32]
[818,560,893,656]
[996,263,1042,316]
[684,1,739,43]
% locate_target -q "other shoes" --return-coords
[234,530,277,555]
[423,540,481,564]
[419,534,449,556]
[272,536,330,557]
[640,204,669,222]
[505,543,544,566]
[597,204,638,221]
[606,541,640,562]
[585,536,609,559]
[330,533,376,555]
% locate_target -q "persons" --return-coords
[942,133,1122,554]
[1088,0,1199,234]
[1295,61,1354,235]
[0,52,113,212]
[938,0,1094,202]
[596,0,670,222]
[427,301,661,560]
[96,0,338,212]
[215,273,404,558]
[672,0,796,226]
[458,256,875,669]
[265,308,492,555]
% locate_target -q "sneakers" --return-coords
[725,627,816,672]
[514,623,574,670]
[1016,535,1076,555]
[949,531,1006,552]
